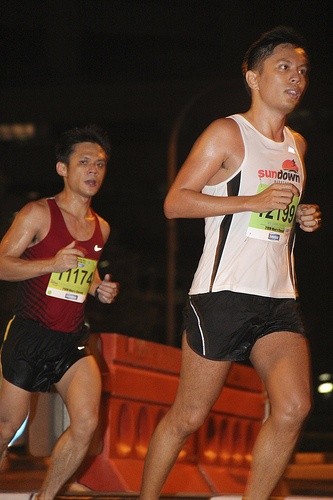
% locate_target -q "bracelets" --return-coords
[94,285,100,303]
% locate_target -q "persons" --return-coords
[140,27,322,500]
[0,122,121,500]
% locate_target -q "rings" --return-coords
[314,219,320,224]
